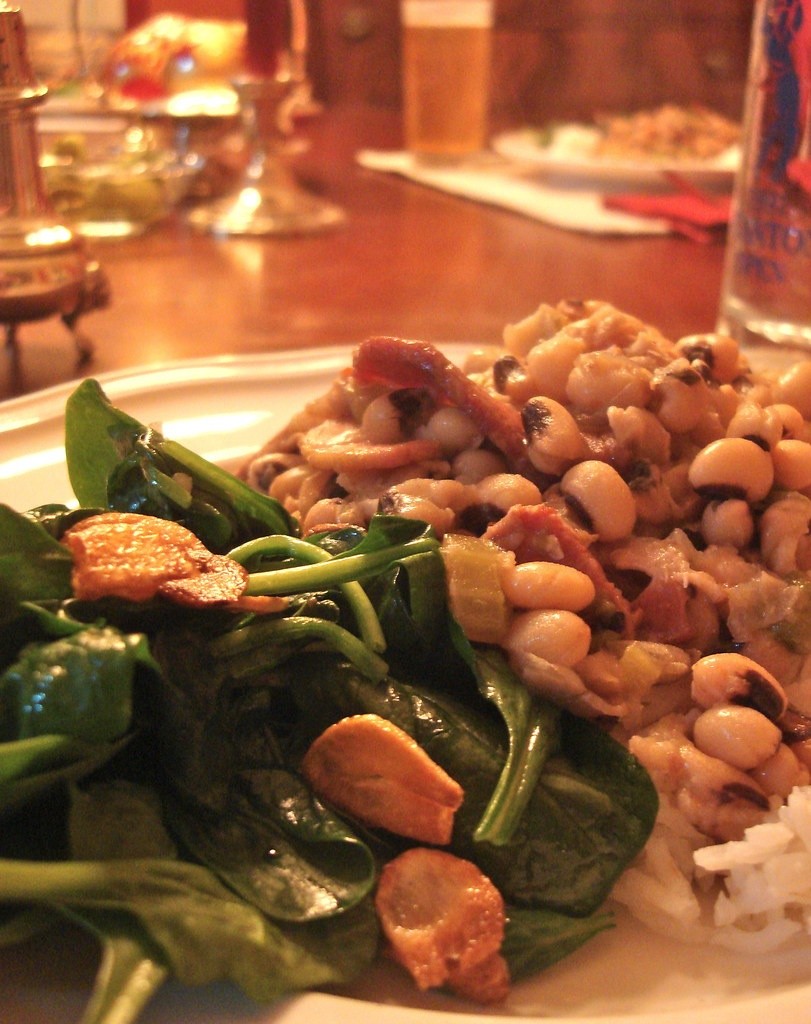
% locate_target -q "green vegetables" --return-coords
[0,378,659,1024]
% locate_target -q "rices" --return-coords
[609,785,811,951]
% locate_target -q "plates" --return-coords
[491,129,740,187]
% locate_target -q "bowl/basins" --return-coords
[39,148,206,237]
[0,344,810,1024]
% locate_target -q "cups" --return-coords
[400,0,494,164]
[713,0,811,385]
